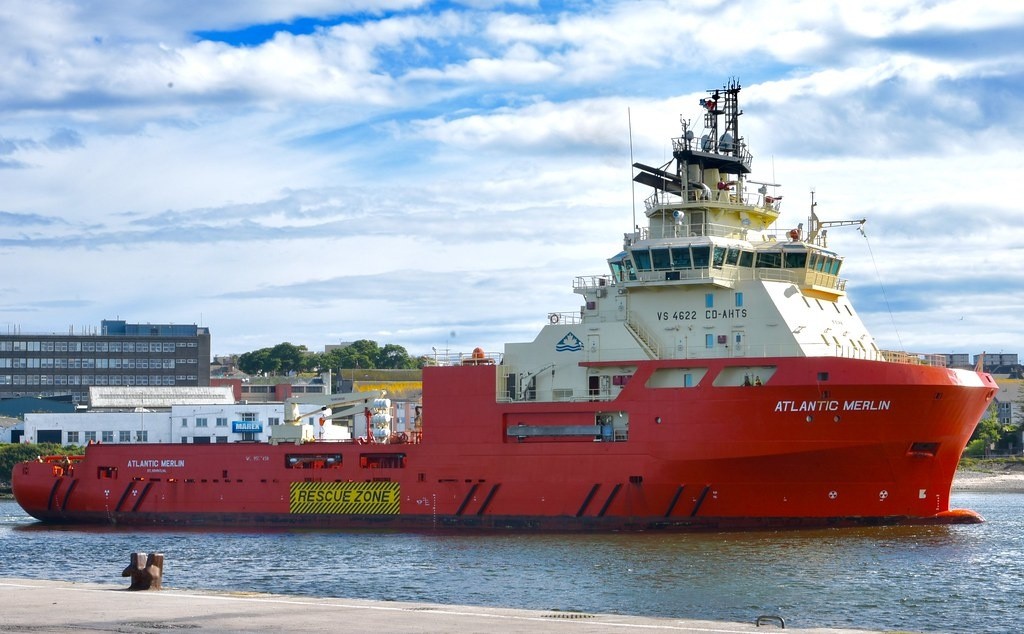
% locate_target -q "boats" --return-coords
[10,73,999,531]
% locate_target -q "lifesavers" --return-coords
[550,314,560,325]
